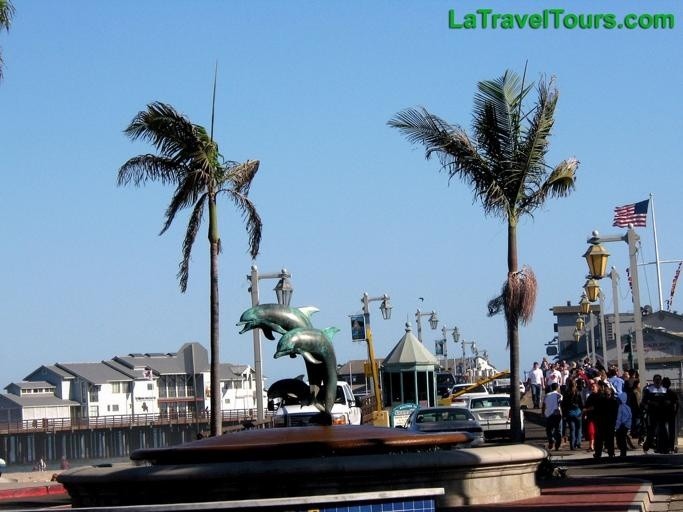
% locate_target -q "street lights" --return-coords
[411,305,490,389]
[359,291,395,411]
[243,265,297,423]
[579,220,648,400]
[570,265,625,375]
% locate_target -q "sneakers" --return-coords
[548,436,594,452]
[631,441,647,454]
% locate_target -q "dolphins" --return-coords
[273,326,342,427]
[267,374,310,407]
[235,304,326,410]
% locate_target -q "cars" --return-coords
[272,369,532,445]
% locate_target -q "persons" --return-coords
[525,357,680,458]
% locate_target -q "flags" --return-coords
[613,199,649,228]
